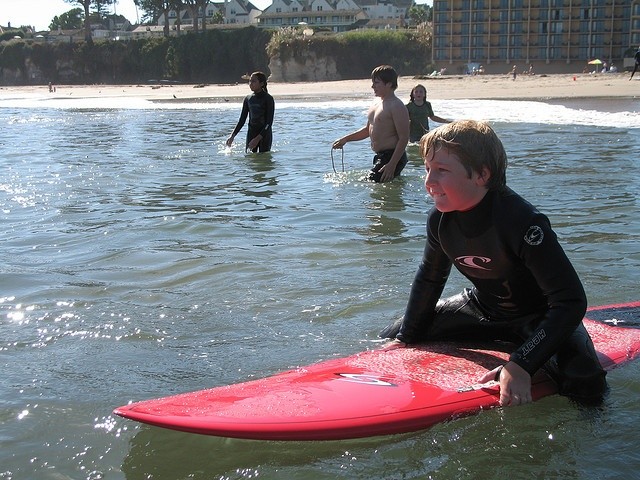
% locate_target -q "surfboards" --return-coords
[113,301,640,440]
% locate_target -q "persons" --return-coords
[334,65,409,183]
[629,46,640,81]
[379,120,607,410]
[404,84,453,143]
[226,72,275,153]
